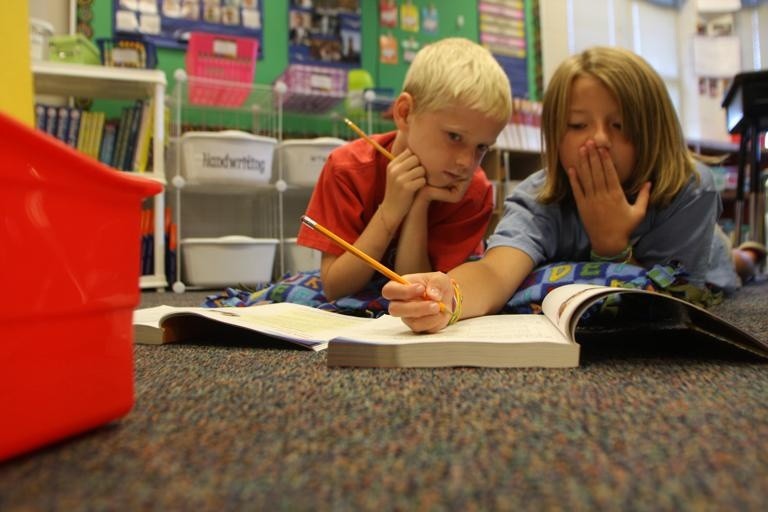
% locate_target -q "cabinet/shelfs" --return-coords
[29,60,167,294]
[170,68,397,293]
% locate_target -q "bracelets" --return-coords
[379,204,394,237]
[591,245,632,265]
[447,278,463,326]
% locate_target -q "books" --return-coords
[131,303,378,353]
[139,207,177,292]
[489,97,546,154]
[34,93,172,173]
[326,284,768,370]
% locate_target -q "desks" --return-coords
[720,69,768,248]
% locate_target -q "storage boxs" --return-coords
[281,136,347,190]
[0,114,164,459]
[284,238,323,273]
[180,130,275,195]
[180,234,280,287]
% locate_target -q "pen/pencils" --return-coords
[300,214,456,316]
[343,117,395,160]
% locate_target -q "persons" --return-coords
[297,36,512,302]
[382,44,768,331]
